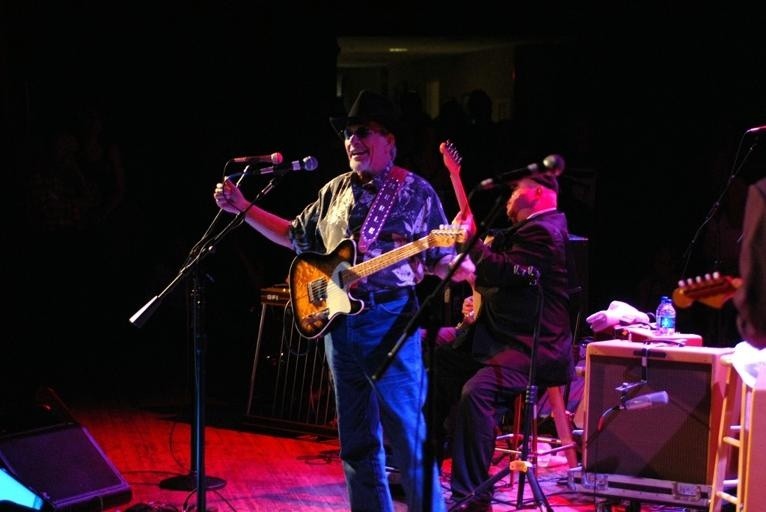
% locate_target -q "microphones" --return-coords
[234,152,283,164]
[260,156,318,174]
[480,154,564,188]
[747,126,766,135]
[256,175,288,200]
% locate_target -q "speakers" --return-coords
[582,339,743,507]
[0,424,131,512]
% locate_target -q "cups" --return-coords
[581,472,594,489]
[596,474,608,491]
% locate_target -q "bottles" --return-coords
[655,296,676,336]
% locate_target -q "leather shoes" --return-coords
[446,499,491,512]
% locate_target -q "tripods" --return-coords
[447,284,551,511]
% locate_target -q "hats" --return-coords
[329,89,410,140]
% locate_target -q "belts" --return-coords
[362,288,409,306]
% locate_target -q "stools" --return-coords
[494,385,578,486]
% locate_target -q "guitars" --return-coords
[288,224,469,338]
[439,139,494,319]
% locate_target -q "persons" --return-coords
[422,172,581,512]
[210,89,480,512]
[734,176,766,354]
